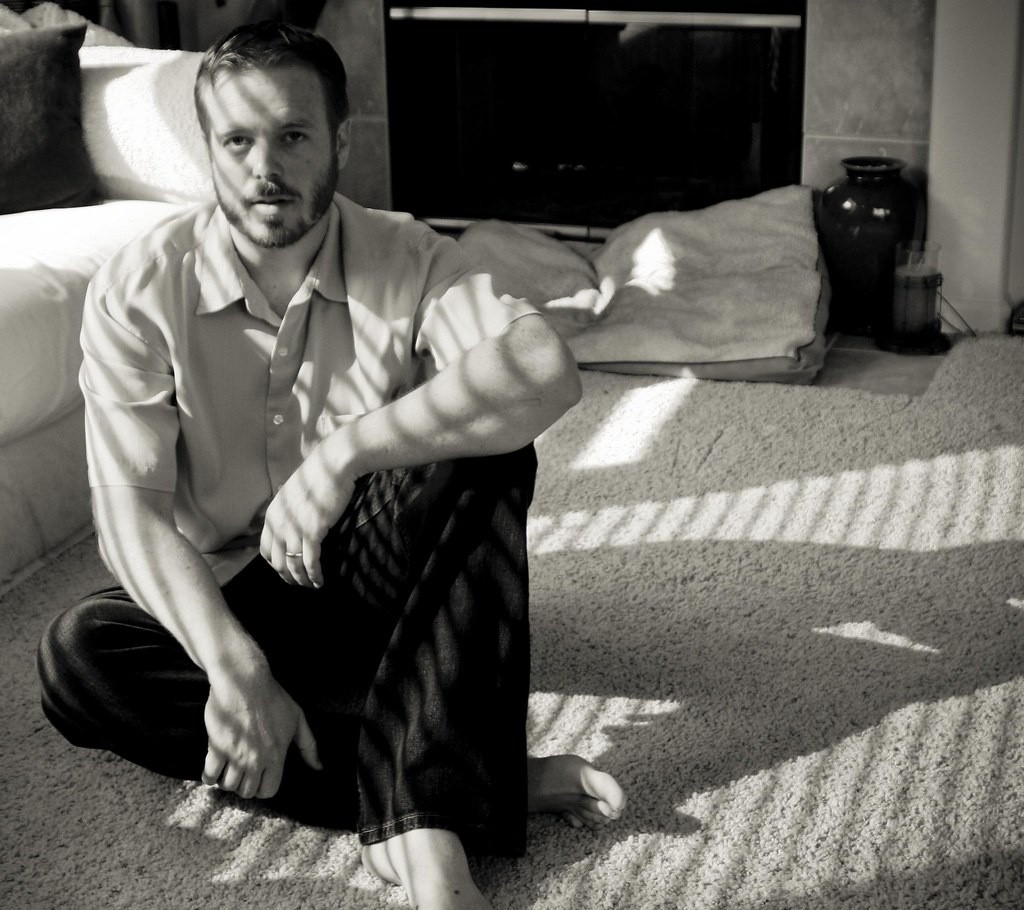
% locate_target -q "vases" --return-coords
[816,155,927,337]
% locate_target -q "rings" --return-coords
[285,552,302,558]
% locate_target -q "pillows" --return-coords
[402,185,832,384]
[0,21,104,215]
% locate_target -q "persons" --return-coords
[36,18,629,910]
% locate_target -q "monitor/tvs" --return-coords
[382,0,807,240]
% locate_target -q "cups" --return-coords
[893,241,942,330]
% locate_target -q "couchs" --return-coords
[0,0,217,597]
